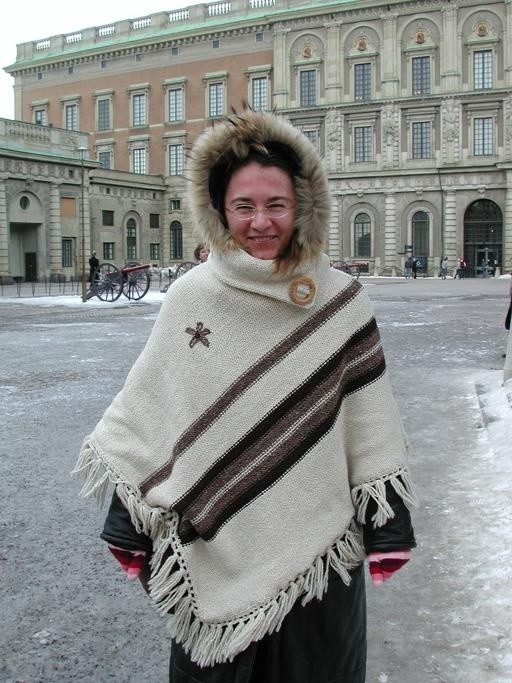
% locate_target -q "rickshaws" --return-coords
[84,262,151,302]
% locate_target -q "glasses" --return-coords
[223,201,297,219]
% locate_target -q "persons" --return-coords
[441,256,449,280]
[88,249,100,288]
[493,260,501,277]
[405,257,419,279]
[194,241,210,262]
[454,255,467,280]
[481,258,490,278]
[74,100,417,683]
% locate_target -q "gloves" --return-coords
[106,542,145,583]
[370,547,412,586]
